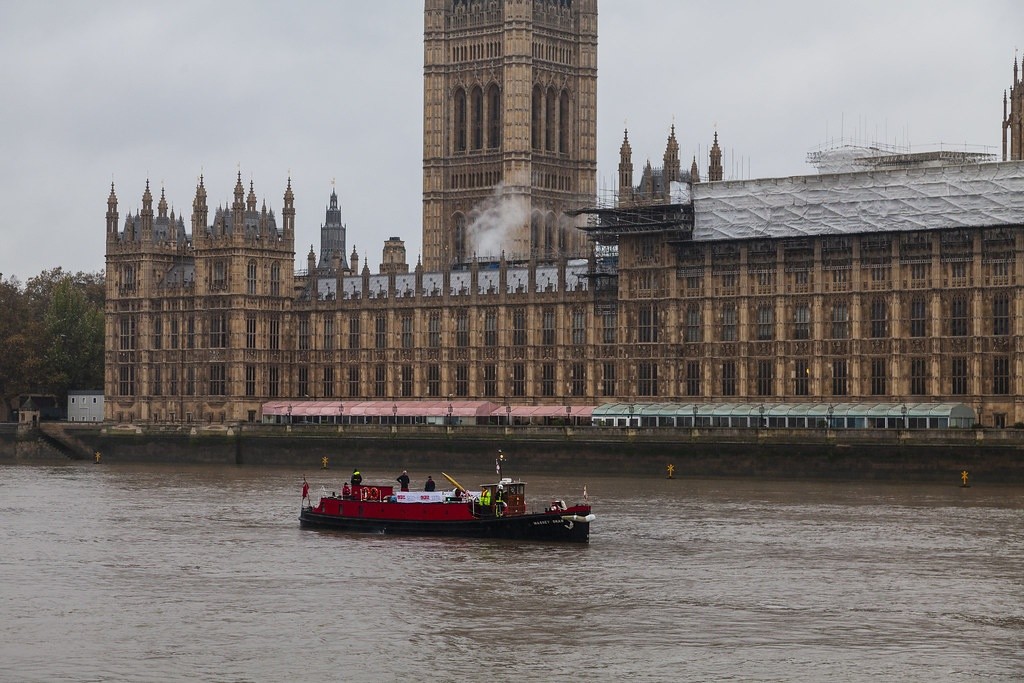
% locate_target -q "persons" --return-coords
[425,476,435,491]
[495,485,504,517]
[397,470,409,488]
[479,486,491,506]
[351,469,362,485]
[343,482,350,494]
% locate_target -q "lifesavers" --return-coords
[361,487,379,499]
[494,502,508,516]
[524,501,526,513]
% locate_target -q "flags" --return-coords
[302,482,309,498]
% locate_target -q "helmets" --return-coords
[497,485,503,490]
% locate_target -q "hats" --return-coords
[403,471,407,473]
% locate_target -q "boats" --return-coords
[298,447,596,543]
